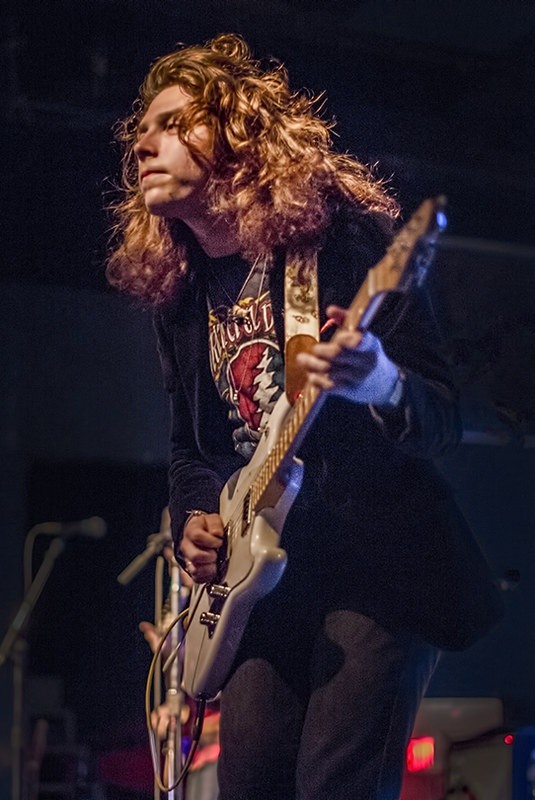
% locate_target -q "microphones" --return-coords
[34,517,107,538]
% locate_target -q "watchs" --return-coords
[381,363,410,419]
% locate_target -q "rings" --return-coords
[185,561,191,572]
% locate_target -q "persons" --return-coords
[112,34,498,800]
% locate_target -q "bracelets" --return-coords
[183,509,209,531]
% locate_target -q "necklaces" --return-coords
[203,247,269,403]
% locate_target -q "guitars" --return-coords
[180,196,453,706]
